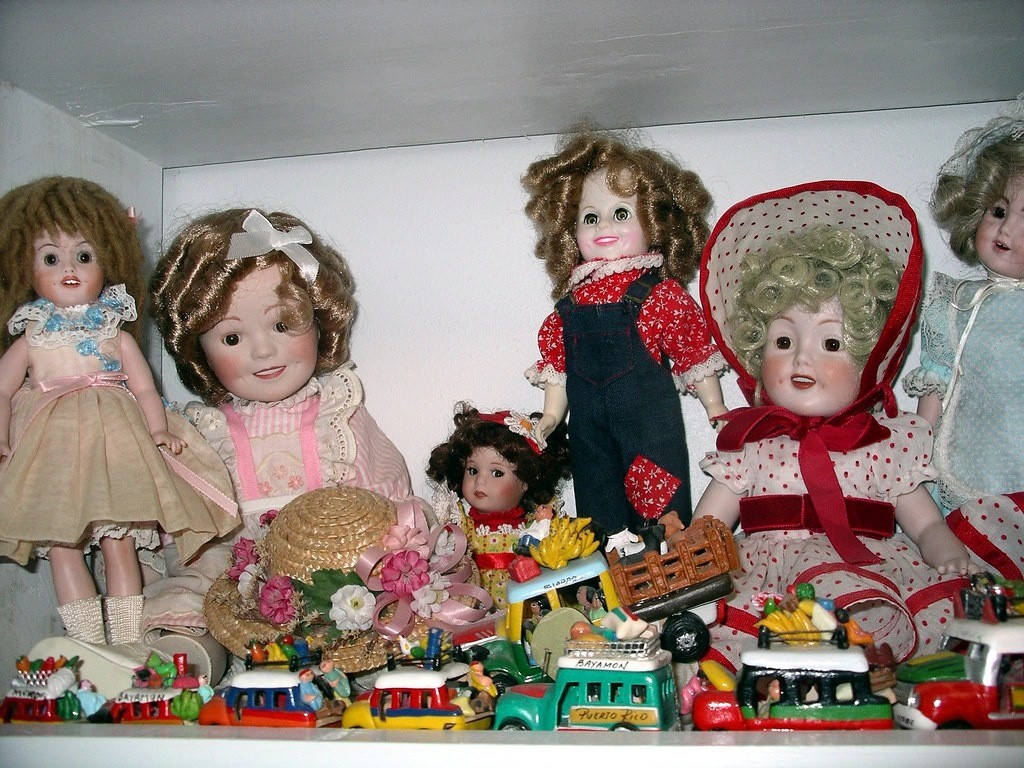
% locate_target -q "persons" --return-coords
[319,660,352,706]
[146,207,414,541]
[576,585,606,623]
[468,661,497,697]
[299,669,322,710]
[684,180,979,662]
[78,680,113,723]
[758,679,780,719]
[903,114,1024,585]
[520,138,732,551]
[198,674,214,704]
[0,174,242,645]
[427,410,570,568]
[522,602,550,643]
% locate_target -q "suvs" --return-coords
[893,595,1024,731]
[492,637,685,733]
[690,622,893,732]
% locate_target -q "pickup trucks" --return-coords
[448,549,735,697]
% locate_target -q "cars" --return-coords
[0,687,66,725]
[85,684,194,726]
[198,647,352,729]
[338,646,494,733]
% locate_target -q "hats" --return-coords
[204,487,481,675]
